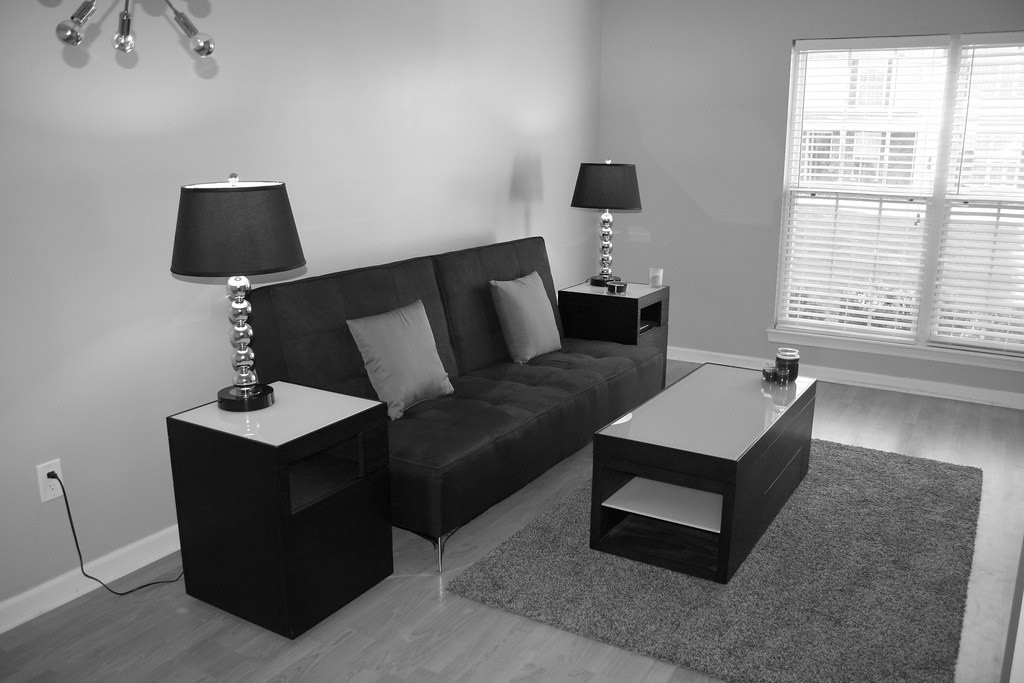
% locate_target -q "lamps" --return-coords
[169,171,308,415]
[571,159,642,285]
[55,0,216,60]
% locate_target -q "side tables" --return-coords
[558,280,670,390]
[166,381,396,642]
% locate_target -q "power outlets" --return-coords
[35,458,64,505]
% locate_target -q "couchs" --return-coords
[247,236,664,575]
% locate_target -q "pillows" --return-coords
[489,271,562,364]
[345,299,454,422]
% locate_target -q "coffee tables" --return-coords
[585,362,817,584]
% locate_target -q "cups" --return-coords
[776,347,800,382]
[763,362,775,379]
[766,366,777,382]
[649,268,663,288]
[777,368,789,386]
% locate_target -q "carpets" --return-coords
[445,438,984,683]
